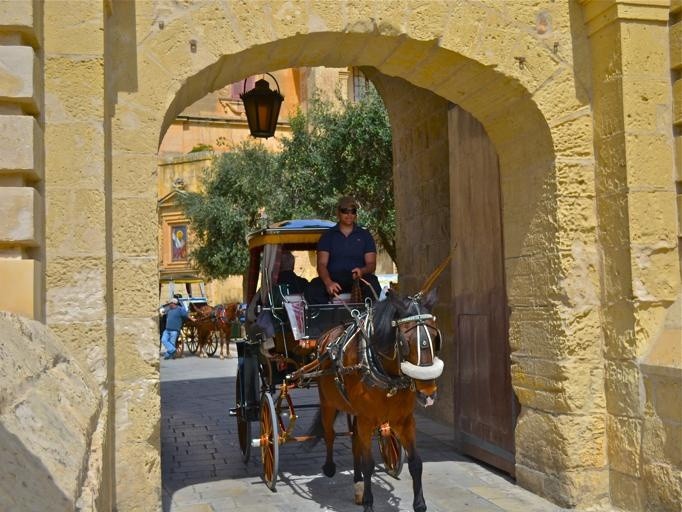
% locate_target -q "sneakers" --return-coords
[163,350,176,360]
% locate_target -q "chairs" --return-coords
[272,285,347,338]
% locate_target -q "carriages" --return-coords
[231,216,446,511]
[159,270,245,360]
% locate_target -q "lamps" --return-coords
[239,72,285,140]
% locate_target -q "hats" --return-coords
[167,298,179,305]
[336,196,360,210]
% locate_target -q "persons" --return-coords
[269,248,310,384]
[160,298,198,360]
[305,196,383,304]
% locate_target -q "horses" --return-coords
[194,301,247,359]
[313,283,441,512]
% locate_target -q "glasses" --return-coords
[340,208,357,215]
